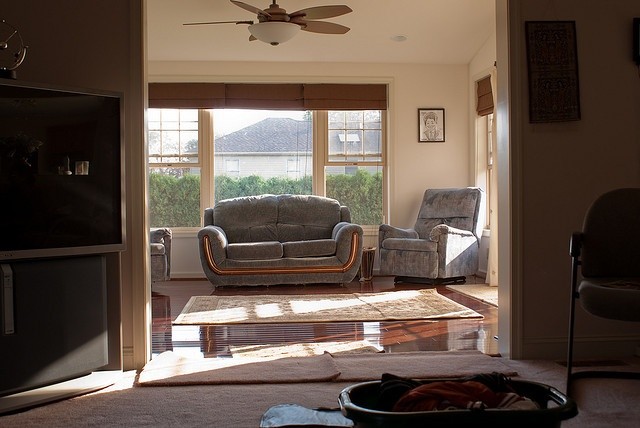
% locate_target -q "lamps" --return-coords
[248,21,301,46]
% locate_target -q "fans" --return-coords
[183,0,353,41]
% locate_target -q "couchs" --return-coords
[197,194,364,288]
[378,187,485,284]
[150,229,174,281]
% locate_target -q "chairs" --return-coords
[564,189,640,397]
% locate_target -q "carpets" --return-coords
[227,339,385,360]
[171,289,484,325]
[137,350,519,386]
[446,285,498,307]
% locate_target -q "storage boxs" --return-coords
[338,377,579,427]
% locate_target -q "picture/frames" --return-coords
[521,17,583,126]
[417,108,446,143]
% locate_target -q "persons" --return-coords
[421,112,443,141]
